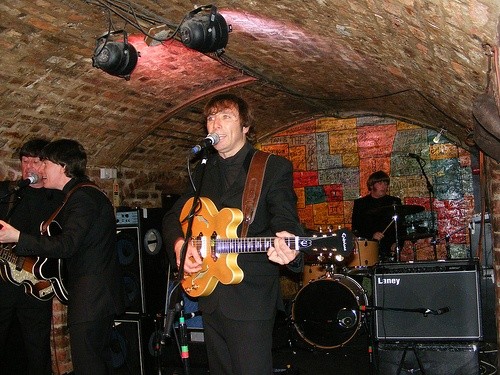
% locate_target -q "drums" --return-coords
[339,237,379,274]
[291,273,369,348]
[302,262,338,286]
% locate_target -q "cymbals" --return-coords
[399,232,441,238]
[372,205,425,216]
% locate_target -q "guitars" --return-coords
[0,218,71,304]
[0,243,56,301]
[174,194,349,299]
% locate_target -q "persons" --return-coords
[0,138,62,375]
[0,138,120,375]
[351,172,406,263]
[160,94,299,375]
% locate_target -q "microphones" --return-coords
[434,307,450,315]
[407,152,420,158]
[14,173,39,191]
[191,133,221,153]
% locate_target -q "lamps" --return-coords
[181,3,230,57]
[90,29,141,80]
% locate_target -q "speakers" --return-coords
[112,318,158,375]
[377,342,479,375]
[373,268,483,342]
[117,226,166,314]
